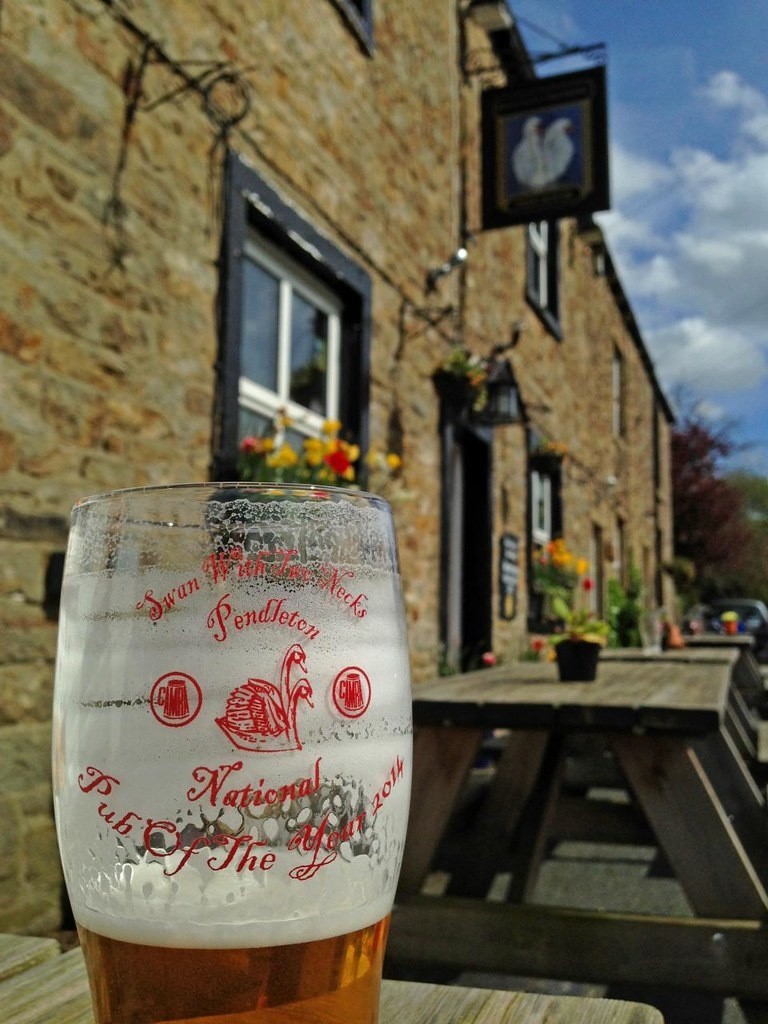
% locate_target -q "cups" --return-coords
[49,481,416,1023]
[639,609,665,655]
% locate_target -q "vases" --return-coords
[724,620,739,635]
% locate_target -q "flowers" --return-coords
[722,610,737,620]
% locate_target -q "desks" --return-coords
[385,661,768,993]
[683,636,768,710]
[599,646,768,763]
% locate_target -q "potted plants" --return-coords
[549,596,610,681]
